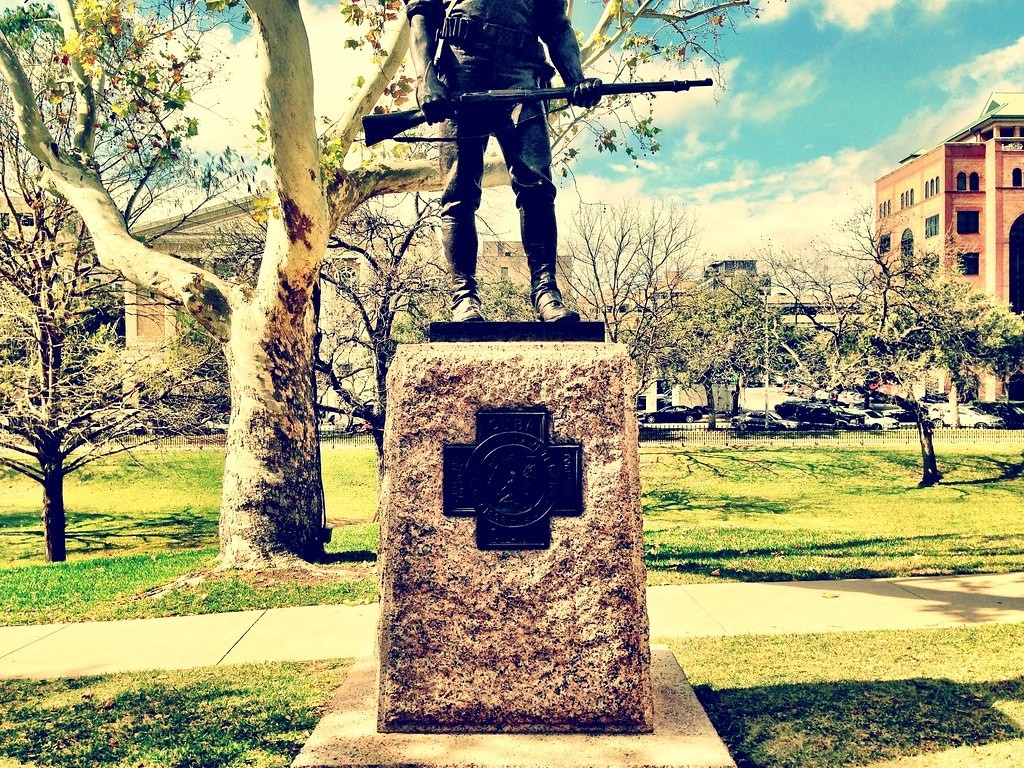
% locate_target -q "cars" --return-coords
[644,405,702,424]
[776,393,1024,431]
[730,410,798,432]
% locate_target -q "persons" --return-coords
[406,0,603,325]
